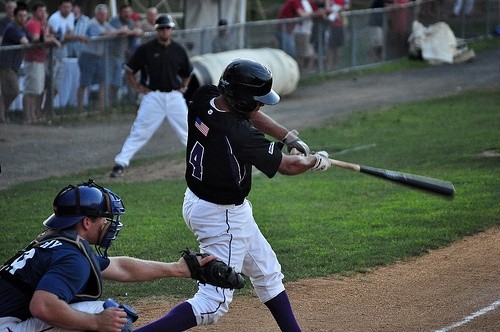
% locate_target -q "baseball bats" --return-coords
[297,154,456,201]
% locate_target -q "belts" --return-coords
[151,88,178,92]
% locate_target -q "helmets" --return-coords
[42,184,126,231]
[154,14,175,28]
[216,57,281,114]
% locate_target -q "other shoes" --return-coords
[109,164,124,177]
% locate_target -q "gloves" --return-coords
[312,150,331,172]
[285,130,310,157]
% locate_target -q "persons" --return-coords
[131,7,160,32]
[22,3,63,123]
[132,59,331,332]
[104,5,140,108]
[0,178,218,332]
[109,13,194,178]
[1,0,19,37]
[364,0,474,64]
[0,6,30,123]
[41,0,90,116]
[274,0,351,73]
[77,3,128,113]
[210,19,236,53]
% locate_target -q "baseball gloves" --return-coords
[180,248,246,291]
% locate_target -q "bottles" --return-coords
[86,27,92,36]
[57,25,62,40]
[327,0,344,23]
[40,23,44,43]
